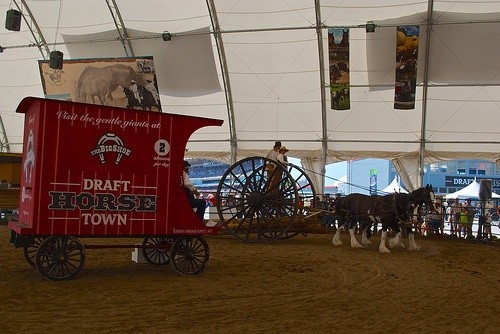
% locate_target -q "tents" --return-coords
[381,178,410,194]
[443,180,500,212]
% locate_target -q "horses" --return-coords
[332,183,437,253]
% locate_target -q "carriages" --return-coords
[216,156,437,253]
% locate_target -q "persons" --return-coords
[298,196,337,227]
[370,174,377,194]
[196,190,243,220]
[264,141,293,198]
[182,159,206,218]
[128,77,161,111]
[411,197,500,241]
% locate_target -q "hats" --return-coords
[184,161,191,167]
[325,193,330,196]
[335,193,342,196]
[436,199,441,204]
[279,146,289,152]
[273,142,281,148]
[298,196,303,198]
